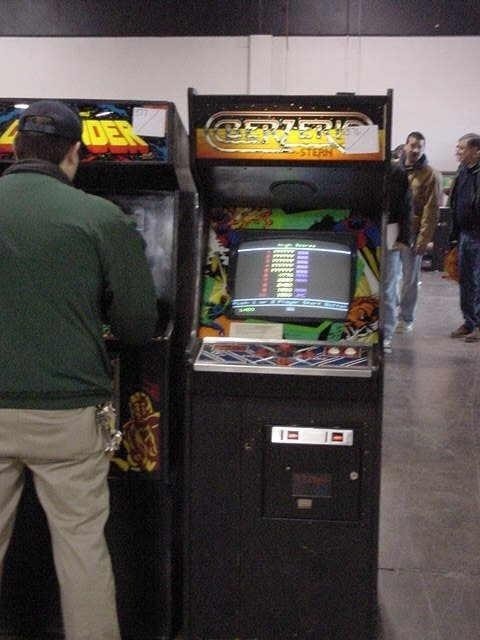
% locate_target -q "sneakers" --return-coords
[450,324,472,338]
[465,326,480,343]
[394,319,417,334]
[382,339,392,354]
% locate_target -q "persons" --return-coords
[382,131,441,355]
[0,100,158,640]
[445,133,480,342]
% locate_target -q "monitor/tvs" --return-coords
[233,238,351,319]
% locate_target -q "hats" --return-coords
[18,99,83,139]
